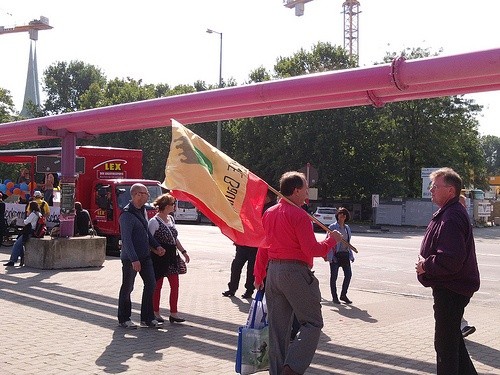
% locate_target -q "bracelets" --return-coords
[182,250,186,254]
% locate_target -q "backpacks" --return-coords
[37,201,46,215]
[32,211,47,238]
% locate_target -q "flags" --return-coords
[157,119,271,248]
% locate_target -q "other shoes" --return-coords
[461,326,476,337]
[333,297,340,304]
[339,295,353,304]
[3,261,14,266]
[241,293,252,298]
[222,289,235,297]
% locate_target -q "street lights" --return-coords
[202,27,223,152]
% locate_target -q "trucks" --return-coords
[0,144,163,257]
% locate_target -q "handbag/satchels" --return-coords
[246,286,268,328]
[88,228,97,234]
[176,254,187,274]
[235,286,270,375]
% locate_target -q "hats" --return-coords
[33,190,42,197]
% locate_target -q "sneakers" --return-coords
[121,320,137,329]
[140,319,163,328]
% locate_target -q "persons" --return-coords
[253,170,343,375]
[323,208,354,304]
[3,202,42,266]
[26,191,50,217]
[118,183,163,329]
[415,167,480,375]
[0,188,59,248]
[75,202,94,236]
[148,192,190,323]
[222,189,310,343]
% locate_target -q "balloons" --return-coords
[0,179,37,204]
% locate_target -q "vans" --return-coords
[173,199,202,225]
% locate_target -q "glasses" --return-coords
[431,184,452,190]
[167,202,175,206]
[136,191,149,195]
[338,211,348,215]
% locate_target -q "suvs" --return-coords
[310,206,338,232]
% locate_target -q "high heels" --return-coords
[154,315,164,322]
[169,315,185,322]
